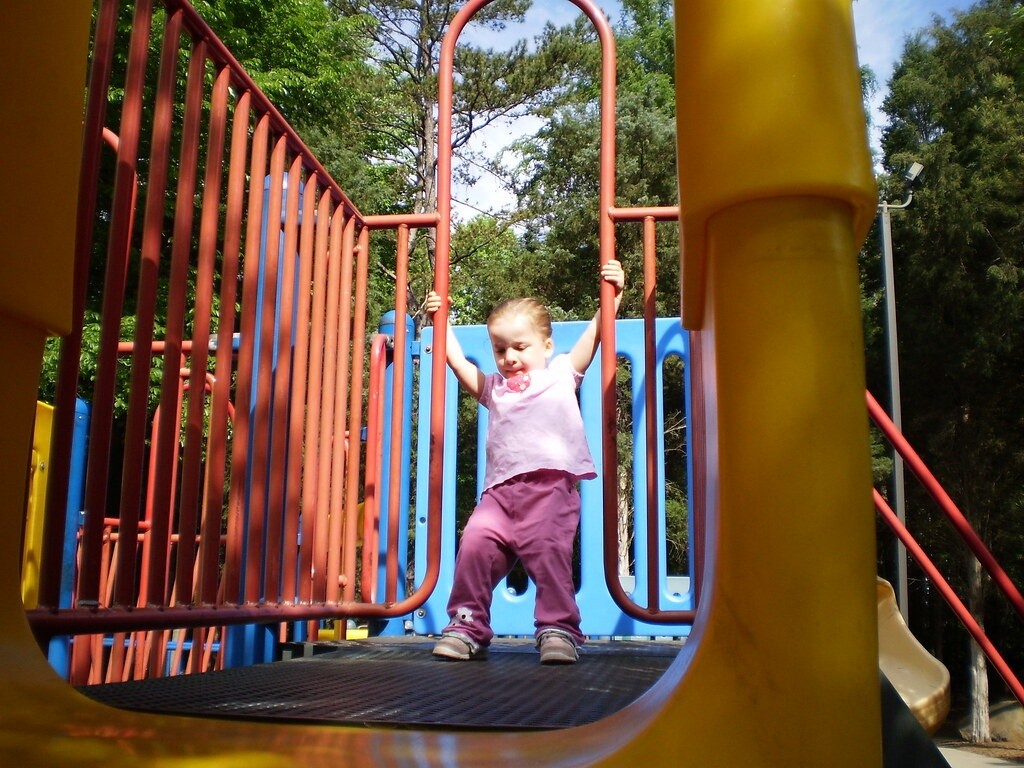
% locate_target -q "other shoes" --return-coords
[540,630,579,662]
[432,636,488,660]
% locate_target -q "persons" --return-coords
[424,259,625,663]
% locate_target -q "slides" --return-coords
[876,573,954,738]
